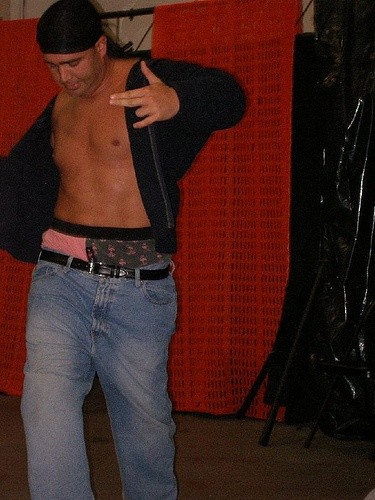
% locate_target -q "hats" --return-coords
[36,0,104,54]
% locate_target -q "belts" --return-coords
[39,251,169,280]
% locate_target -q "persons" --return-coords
[0,0,248,500]
